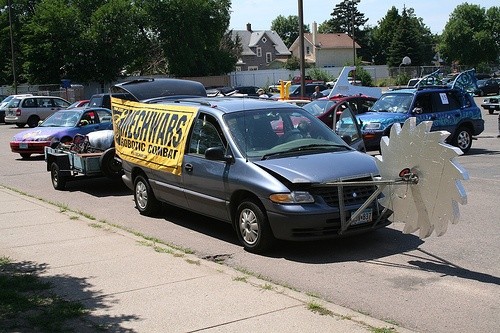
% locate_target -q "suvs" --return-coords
[334,84,485,155]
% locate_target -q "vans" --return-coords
[112,75,394,253]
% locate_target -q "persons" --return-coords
[256,88,270,99]
[278,121,311,143]
[311,86,323,99]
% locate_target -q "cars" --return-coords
[1,73,500,190]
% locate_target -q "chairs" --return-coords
[84,115,93,124]
[247,119,268,149]
